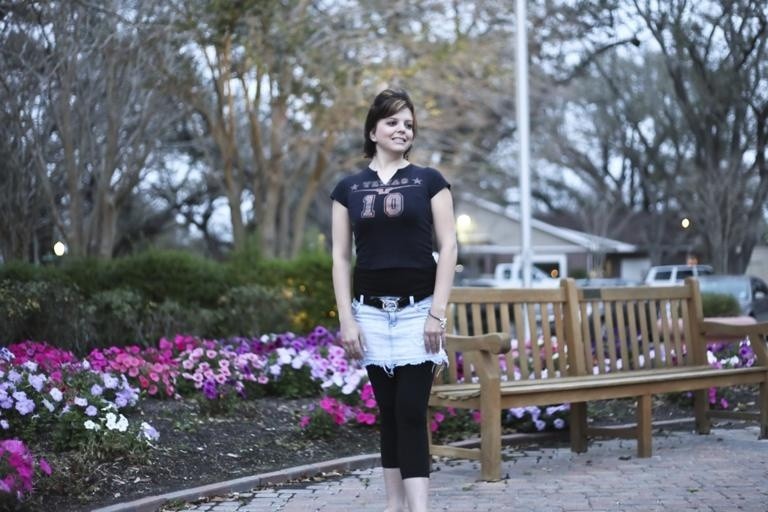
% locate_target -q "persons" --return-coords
[328,87,460,511]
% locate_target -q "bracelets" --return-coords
[425,308,449,328]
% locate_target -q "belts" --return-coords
[352,286,434,309]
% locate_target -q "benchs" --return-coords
[424,272,768,484]
